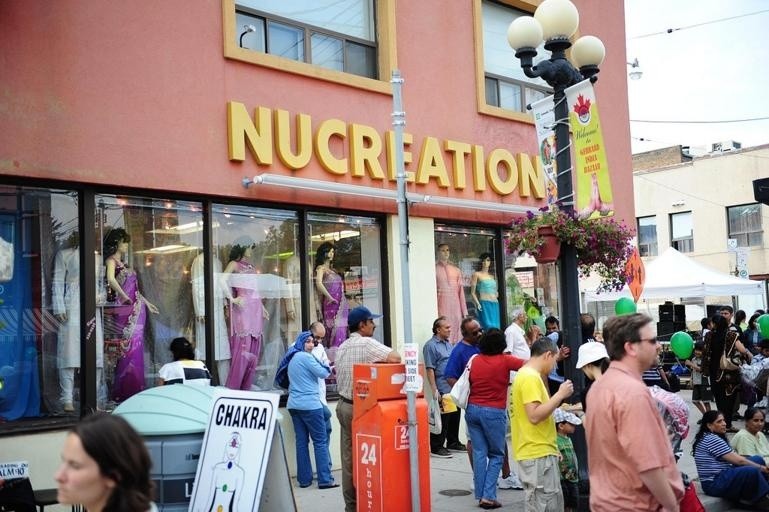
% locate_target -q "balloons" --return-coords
[615,298,636,315]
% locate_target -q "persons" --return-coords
[578,174,614,221]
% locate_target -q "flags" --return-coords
[623,246,646,304]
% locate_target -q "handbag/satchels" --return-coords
[720,353,741,371]
[451,353,477,410]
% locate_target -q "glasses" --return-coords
[471,328,484,337]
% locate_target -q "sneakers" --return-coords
[726,425,740,434]
[430,442,524,509]
[300,480,341,490]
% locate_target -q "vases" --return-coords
[580,238,601,266]
[528,224,561,265]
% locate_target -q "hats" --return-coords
[576,342,608,372]
[347,307,382,325]
[554,408,582,426]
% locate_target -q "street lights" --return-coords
[509,0,605,512]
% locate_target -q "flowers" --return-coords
[504,202,589,256]
[577,215,638,294]
